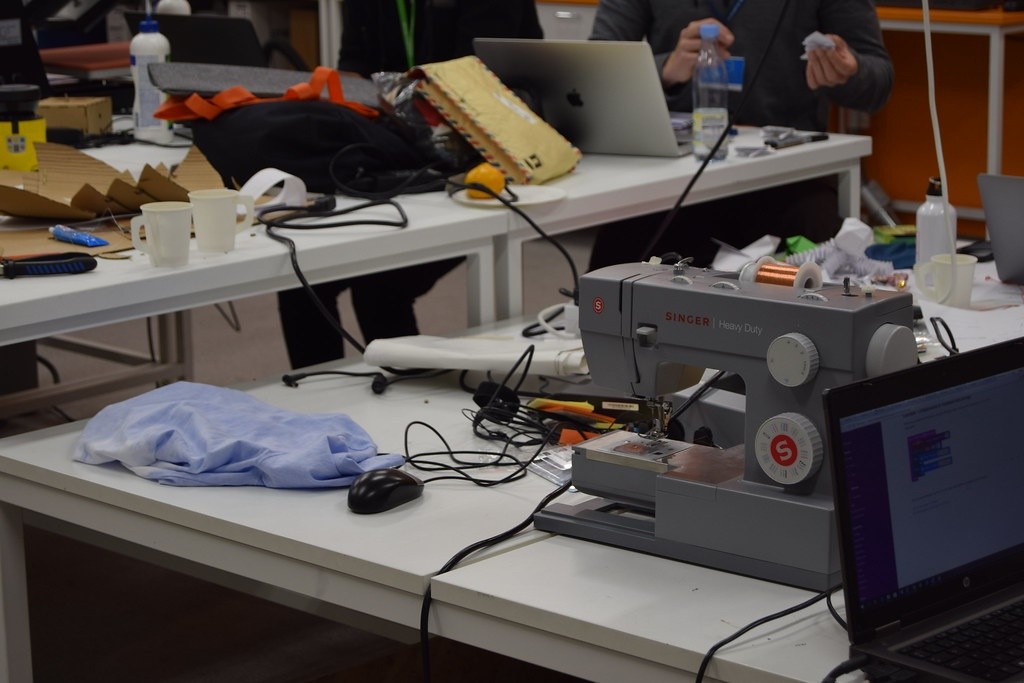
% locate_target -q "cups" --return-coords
[931,252,978,306]
[131,189,252,267]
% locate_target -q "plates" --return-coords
[452,186,564,205]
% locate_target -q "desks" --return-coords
[1,241,1023,683]
[0,128,873,444]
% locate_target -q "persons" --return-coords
[583,0,892,265]
[276,0,533,371]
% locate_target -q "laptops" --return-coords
[122,9,268,68]
[472,37,695,158]
[823,336,1024,683]
[978,174,1024,286]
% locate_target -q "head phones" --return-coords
[471,344,536,442]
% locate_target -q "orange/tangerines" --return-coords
[465,162,505,198]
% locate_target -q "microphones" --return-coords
[282,370,388,394]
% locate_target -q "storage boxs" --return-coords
[37,98,112,136]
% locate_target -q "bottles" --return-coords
[916,179,955,295]
[130,19,171,148]
[693,23,728,161]
[158,0,191,14]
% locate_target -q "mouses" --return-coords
[346,467,425,514]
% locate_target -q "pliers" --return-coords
[0,251,97,279]
